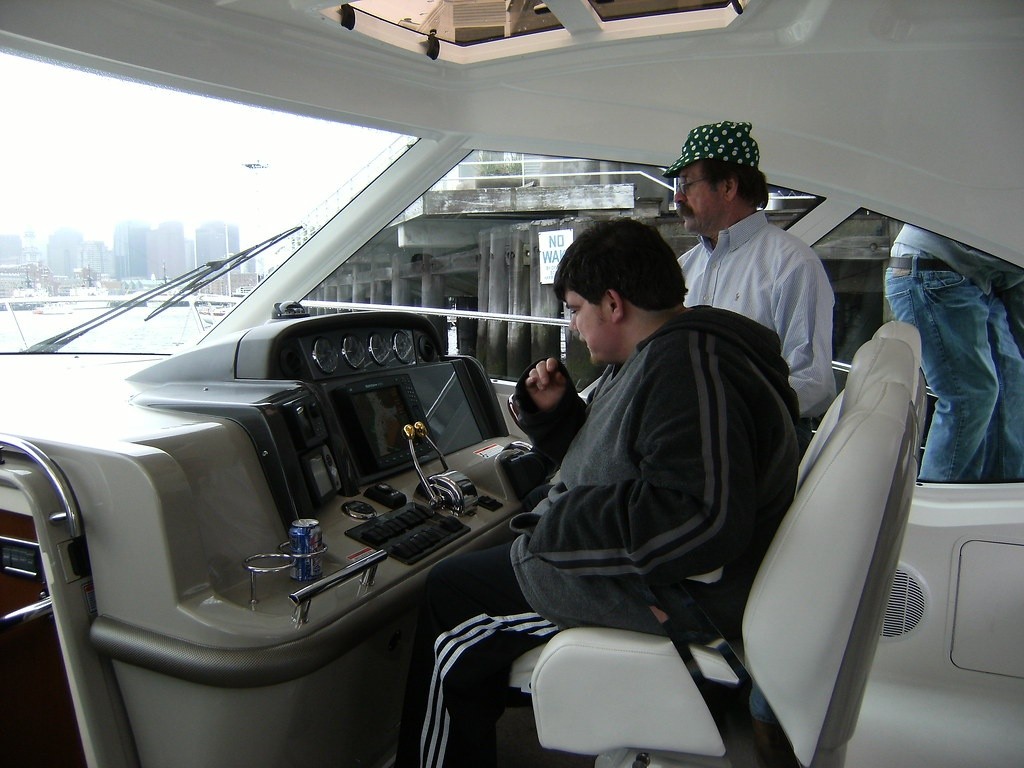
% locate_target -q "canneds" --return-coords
[288,518,323,582]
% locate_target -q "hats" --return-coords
[661,121,759,178]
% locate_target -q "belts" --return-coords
[887,257,957,271]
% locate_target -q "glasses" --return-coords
[674,178,706,195]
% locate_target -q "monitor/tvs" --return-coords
[352,385,417,458]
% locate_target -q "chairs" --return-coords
[510,319,929,768]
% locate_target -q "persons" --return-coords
[662,121,837,463]
[393,218,800,768]
[749,680,800,768]
[884,223,1024,484]
